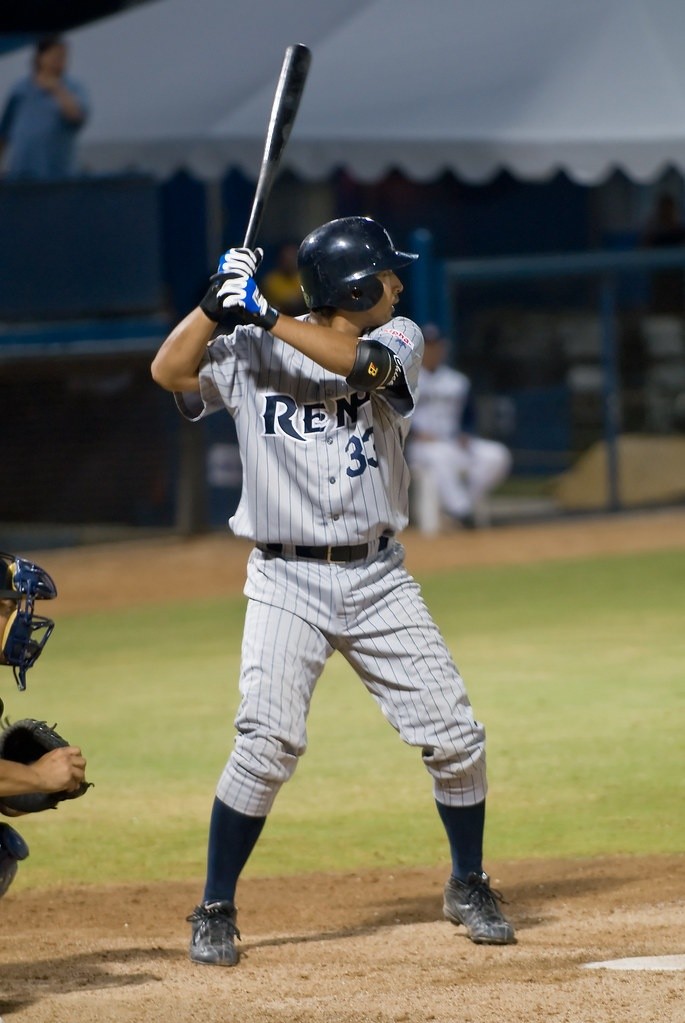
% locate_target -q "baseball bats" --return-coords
[233,44,312,249]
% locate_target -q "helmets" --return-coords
[296,216,419,313]
[0,557,20,601]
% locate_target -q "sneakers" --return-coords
[442,870,516,945]
[186,896,241,966]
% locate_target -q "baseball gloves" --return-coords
[0,715,96,816]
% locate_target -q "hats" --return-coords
[418,323,447,345]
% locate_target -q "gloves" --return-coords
[198,247,279,333]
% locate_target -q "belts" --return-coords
[265,536,389,564]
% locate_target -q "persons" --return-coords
[0,553,94,900]
[150,217,518,967]
[259,228,513,540]
[0,34,92,184]
